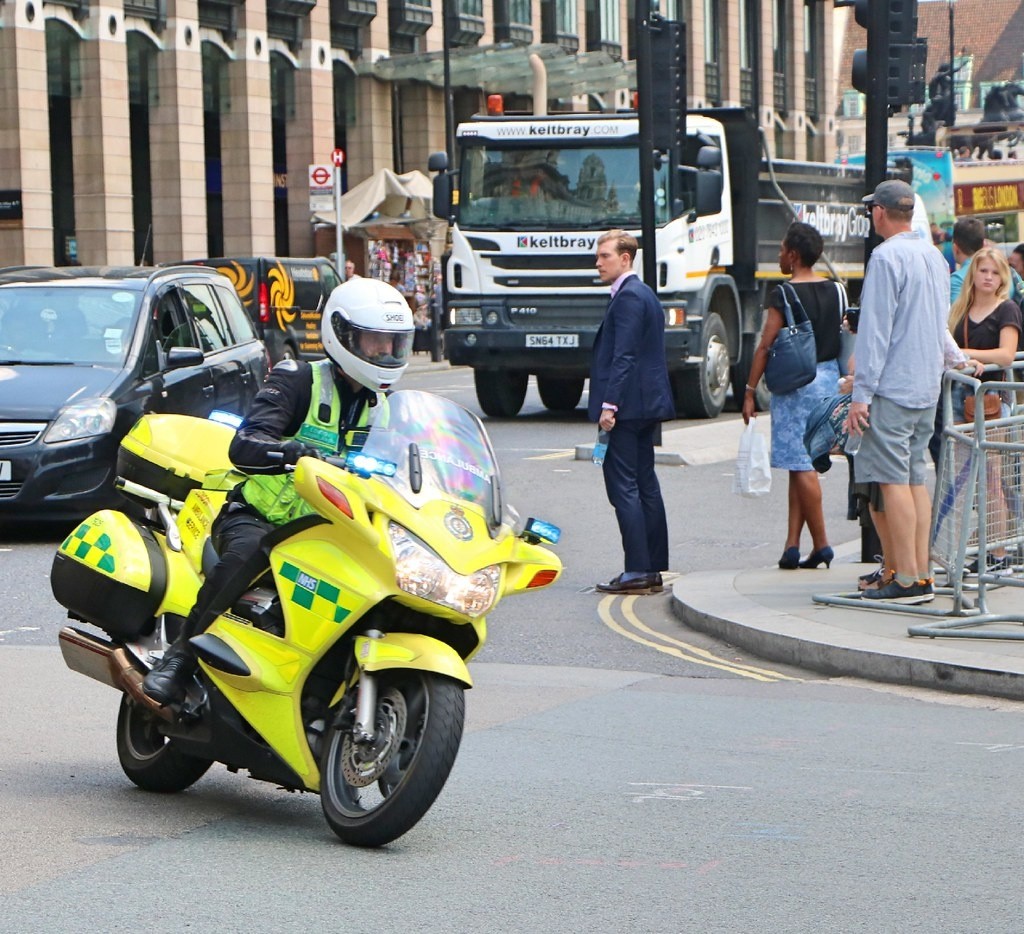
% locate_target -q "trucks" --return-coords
[425,0,917,417]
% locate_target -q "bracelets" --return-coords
[745,384,756,391]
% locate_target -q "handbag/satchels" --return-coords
[765,282,817,395]
[835,280,859,376]
[732,416,772,498]
[964,394,1002,422]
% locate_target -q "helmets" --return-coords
[321,278,415,393]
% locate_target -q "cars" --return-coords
[0,265,270,537]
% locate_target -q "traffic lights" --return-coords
[850,0,873,97]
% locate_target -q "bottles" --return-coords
[591,428,610,467]
[844,417,867,454]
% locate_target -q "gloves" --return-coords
[280,441,321,469]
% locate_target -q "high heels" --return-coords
[800,546,834,568]
[778,546,800,569]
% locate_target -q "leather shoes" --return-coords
[142,647,199,703]
[595,572,663,594]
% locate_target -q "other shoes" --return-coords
[963,553,1013,577]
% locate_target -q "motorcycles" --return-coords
[49,388,570,851]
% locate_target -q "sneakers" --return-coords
[917,577,935,601]
[859,571,923,606]
[861,571,897,590]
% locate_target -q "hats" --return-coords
[860,179,915,211]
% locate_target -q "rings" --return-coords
[859,416,863,421]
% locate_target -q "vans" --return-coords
[154,255,347,374]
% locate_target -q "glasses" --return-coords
[867,201,885,212]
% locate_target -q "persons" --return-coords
[144,273,416,705]
[921,59,969,131]
[847,178,951,604]
[742,222,853,570]
[381,262,407,298]
[500,152,553,218]
[343,261,365,283]
[955,147,1019,164]
[838,216,1024,591]
[586,230,673,596]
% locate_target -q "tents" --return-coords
[311,170,450,248]
